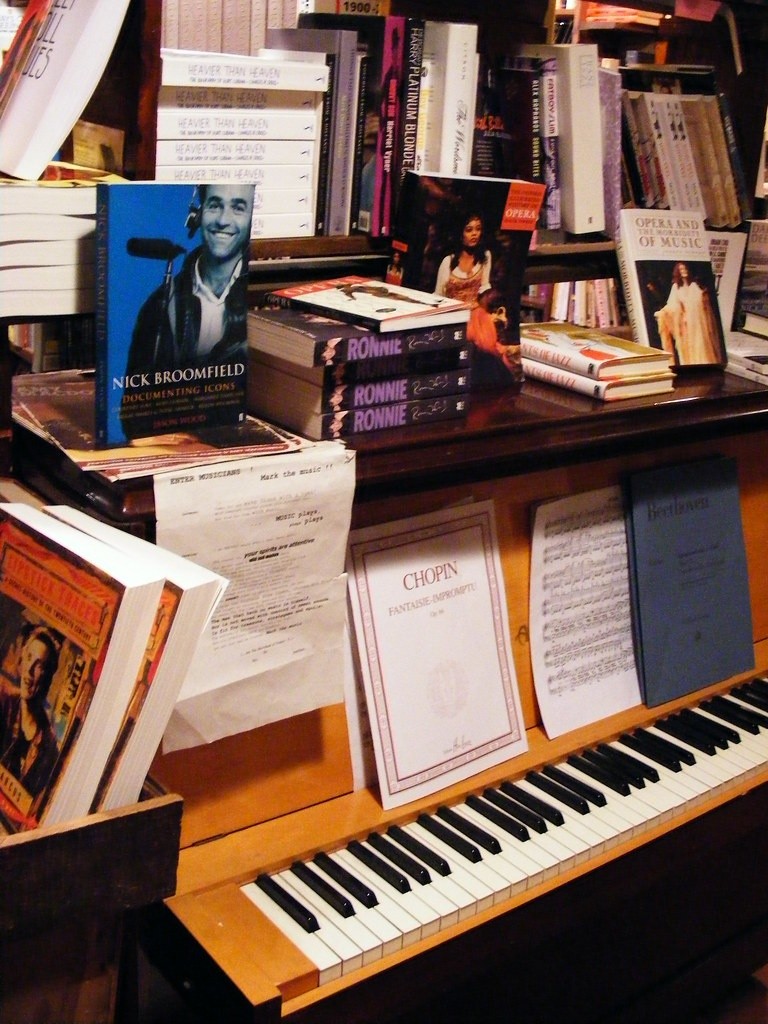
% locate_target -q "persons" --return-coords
[1,626,60,799]
[429,209,514,386]
[358,113,380,216]
[654,260,721,367]
[384,249,405,286]
[119,183,251,447]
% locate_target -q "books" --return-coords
[1,0,766,845]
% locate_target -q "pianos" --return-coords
[67,358,768,1023]
